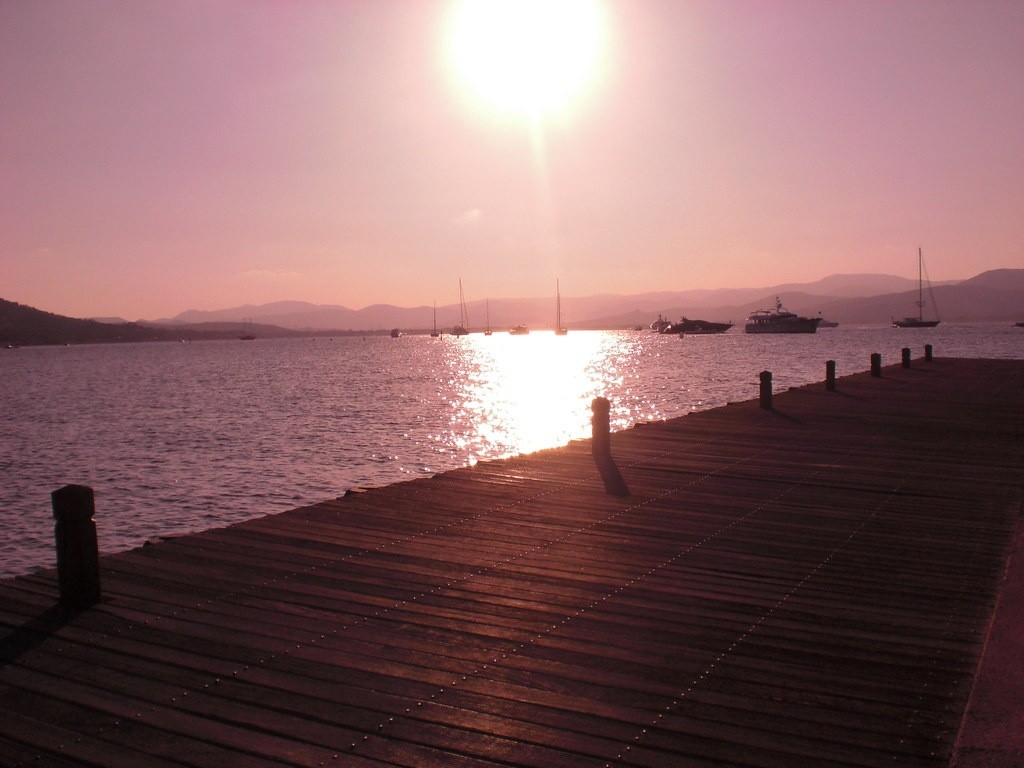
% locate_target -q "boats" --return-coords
[509,323,530,336]
[634,310,735,335]
[743,294,823,336]
[818,319,841,328]
[390,328,402,338]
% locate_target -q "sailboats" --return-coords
[554,276,570,336]
[484,298,494,335]
[450,277,470,334]
[890,247,943,328]
[242,316,257,340]
[431,300,440,337]
